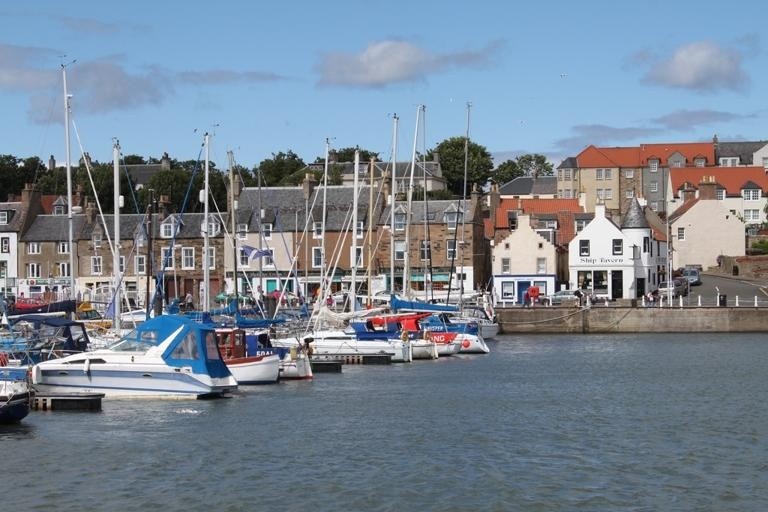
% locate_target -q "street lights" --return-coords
[657,197,675,306]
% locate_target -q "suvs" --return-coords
[538,288,594,308]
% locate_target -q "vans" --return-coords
[657,280,685,299]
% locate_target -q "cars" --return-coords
[673,277,692,294]
[681,269,700,285]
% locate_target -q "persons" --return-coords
[647,290,655,307]
[522,288,530,310]
[184,291,195,311]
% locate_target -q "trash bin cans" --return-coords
[631,299,637,308]
[720,295,727,306]
[733,266,739,276]
